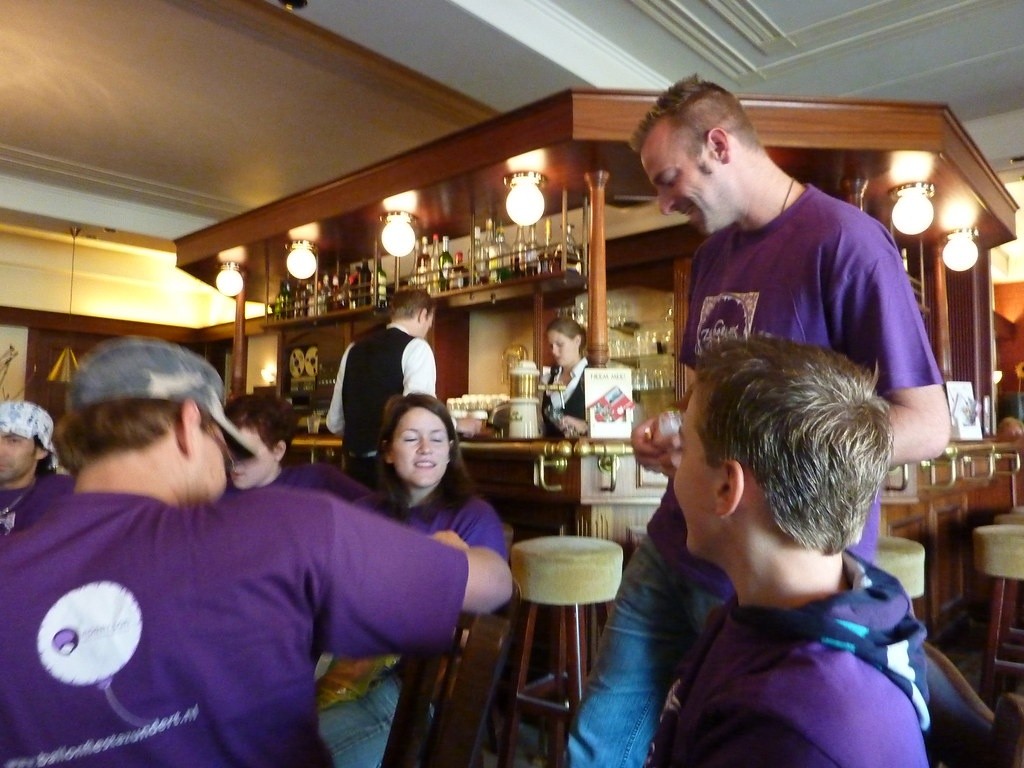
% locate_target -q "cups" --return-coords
[446,393,511,414]
[307,413,321,434]
[569,297,675,391]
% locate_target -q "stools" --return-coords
[497,537,623,767]
[977,504,1023,707]
[871,536,925,598]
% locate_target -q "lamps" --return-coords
[287,241,317,280]
[46,225,78,380]
[380,211,415,257]
[216,261,243,297]
[503,171,548,227]
[890,182,934,235]
[941,228,979,272]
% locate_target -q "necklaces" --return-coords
[783,177,794,215]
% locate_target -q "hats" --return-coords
[0,400,55,454]
[66,335,256,461]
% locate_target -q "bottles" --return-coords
[658,409,687,467]
[405,217,579,298]
[273,250,387,320]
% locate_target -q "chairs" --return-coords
[381,523,520,766]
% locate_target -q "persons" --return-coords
[563,72,951,768]
[323,286,487,490]
[537,316,590,437]
[643,338,930,768]
[0,334,511,768]
